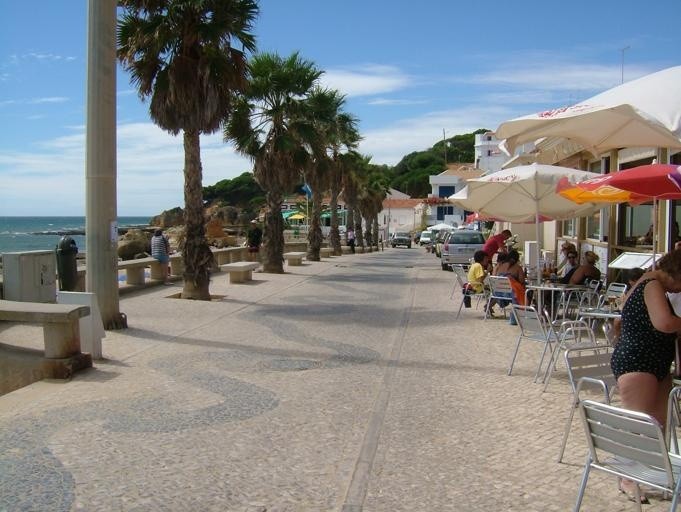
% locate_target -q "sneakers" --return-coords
[164,282,175,286]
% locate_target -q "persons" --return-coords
[435,231,440,240]
[467,243,600,326]
[609,250,680,504]
[346,227,355,253]
[246,220,263,271]
[480,229,513,274]
[613,267,645,343]
[151,229,173,285]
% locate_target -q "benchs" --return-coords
[355,245,377,254]
[0,299,91,380]
[341,246,351,254]
[320,248,334,258]
[117,240,330,286]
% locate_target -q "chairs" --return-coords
[450,263,634,393]
[557,344,619,461]
[572,398,681,511]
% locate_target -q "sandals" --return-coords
[619,477,649,504]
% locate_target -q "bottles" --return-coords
[542,263,555,278]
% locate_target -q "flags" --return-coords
[302,183,312,199]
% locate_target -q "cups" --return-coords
[608,293,624,312]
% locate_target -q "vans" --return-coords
[414,230,486,270]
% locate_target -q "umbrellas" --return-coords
[447,162,609,282]
[555,159,681,272]
[425,222,457,230]
[287,214,306,224]
[494,65,681,158]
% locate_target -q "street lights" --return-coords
[621,45,631,84]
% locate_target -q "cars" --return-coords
[392,231,411,248]
